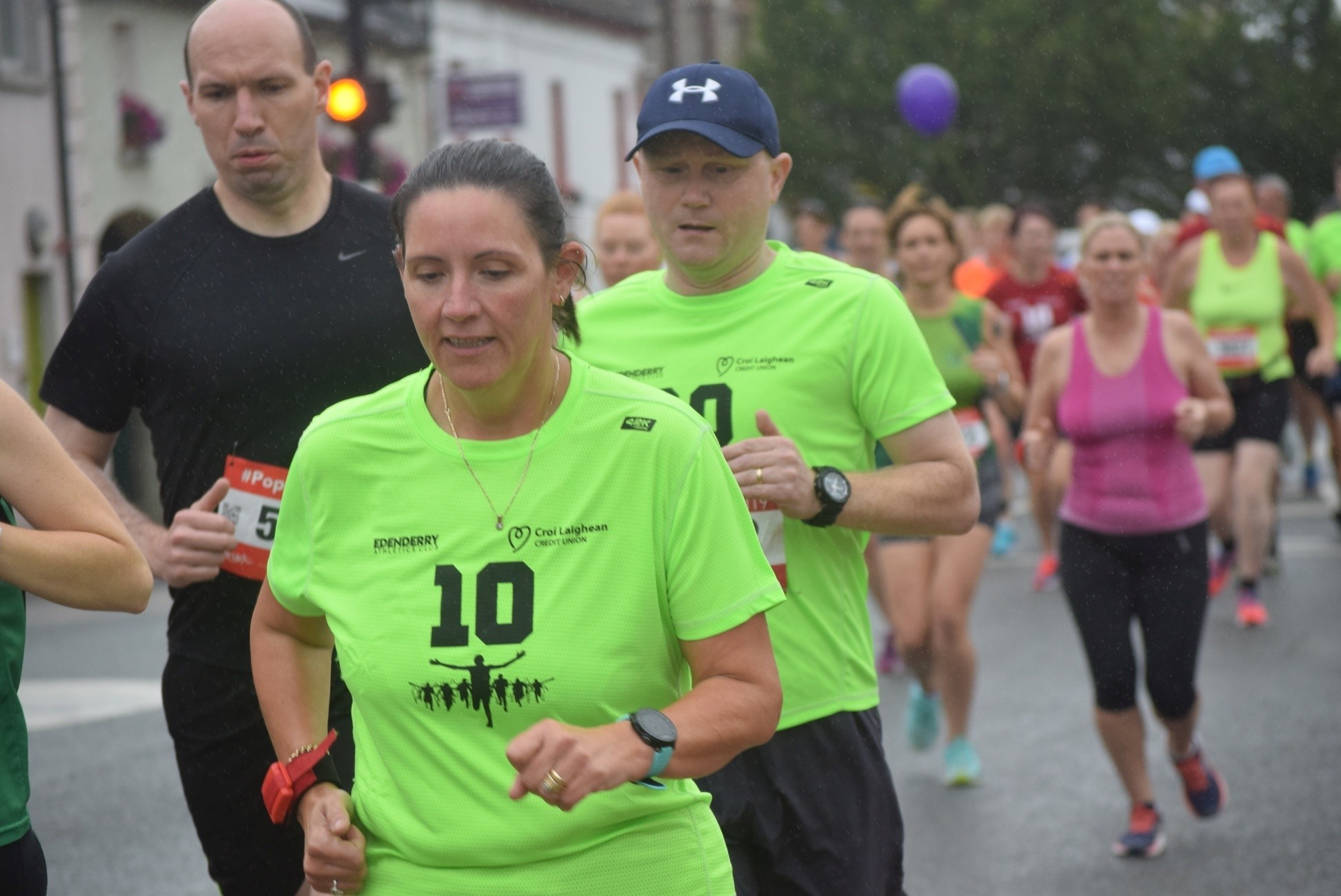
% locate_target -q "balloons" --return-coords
[892,62,959,138]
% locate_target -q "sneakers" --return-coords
[1109,803,1166,858]
[907,686,939,749]
[1207,550,1236,594]
[1235,593,1266,626]
[942,737,982,786]
[1165,738,1228,819]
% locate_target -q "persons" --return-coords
[868,181,1029,791]
[37,0,432,896]
[1157,173,1338,628]
[764,143,1341,791]
[0,373,153,896]
[1014,207,1238,860]
[247,137,789,896]
[590,191,665,288]
[553,60,981,896]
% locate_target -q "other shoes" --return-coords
[1036,556,1060,592]
[992,525,1020,556]
[880,634,906,678]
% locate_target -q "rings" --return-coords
[329,879,344,896]
[539,769,568,797]
[756,468,763,485]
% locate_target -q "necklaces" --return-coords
[439,346,559,530]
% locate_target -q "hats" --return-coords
[1191,145,1244,182]
[624,60,781,163]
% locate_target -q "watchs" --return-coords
[802,465,851,528]
[614,707,678,790]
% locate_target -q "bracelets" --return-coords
[989,369,1010,396]
[259,718,352,825]
